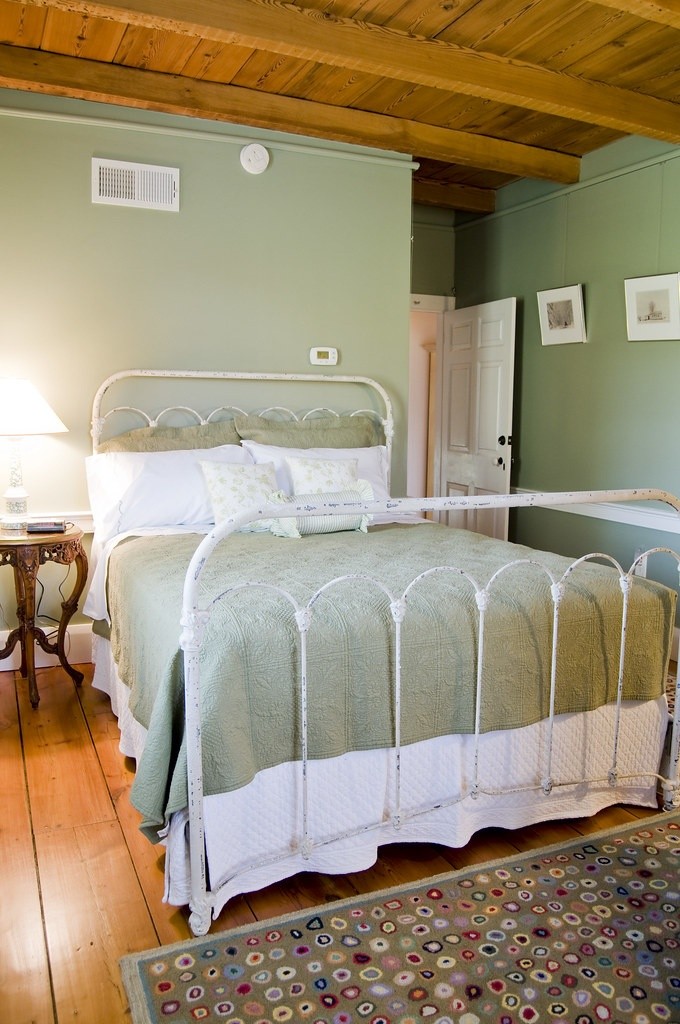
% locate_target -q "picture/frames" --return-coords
[624,272,680,342]
[536,283,587,346]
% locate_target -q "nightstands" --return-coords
[1,519,88,708]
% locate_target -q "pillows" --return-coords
[266,484,376,540]
[97,419,242,457]
[285,456,360,494]
[200,460,279,535]
[233,415,386,450]
[84,445,259,540]
[245,438,392,517]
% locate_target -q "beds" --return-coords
[84,371,680,935]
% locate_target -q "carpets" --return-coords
[118,803,680,1024]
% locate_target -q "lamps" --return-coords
[0,373,69,536]
